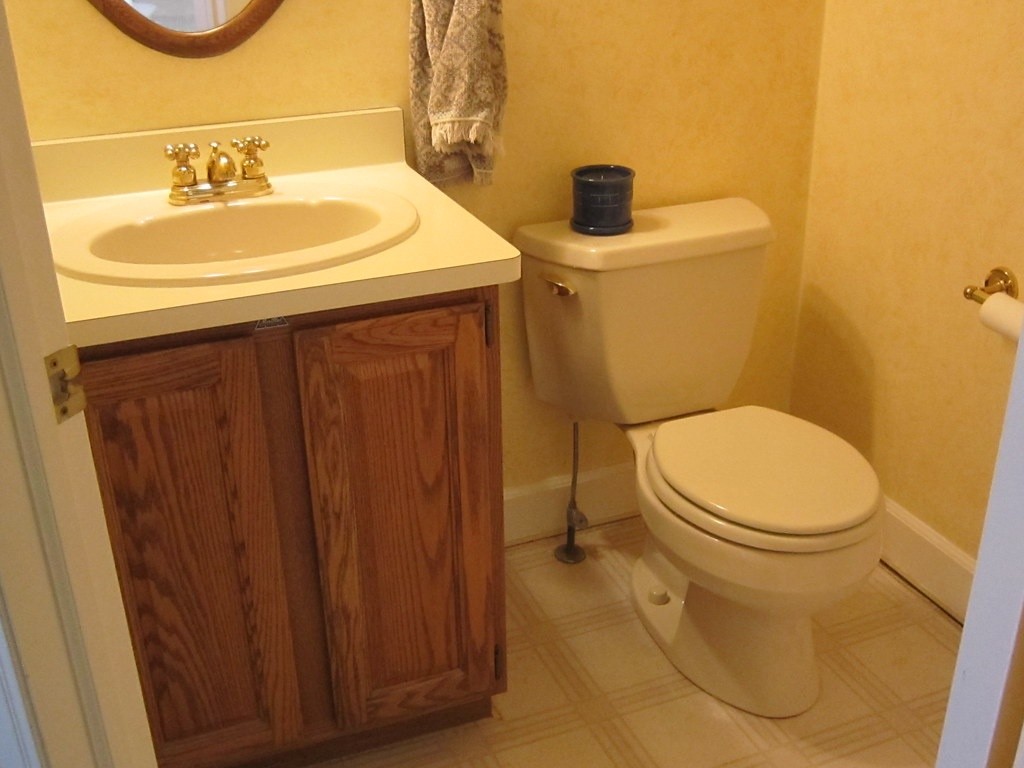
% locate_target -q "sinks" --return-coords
[50,192,421,283]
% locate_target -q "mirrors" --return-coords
[88,0,284,60]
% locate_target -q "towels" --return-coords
[408,0,507,189]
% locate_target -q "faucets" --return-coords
[164,134,274,206]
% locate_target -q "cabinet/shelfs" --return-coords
[41,163,524,768]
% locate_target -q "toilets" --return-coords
[513,198,886,718]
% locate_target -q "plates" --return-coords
[569,218,633,235]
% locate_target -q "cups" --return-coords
[571,165,635,228]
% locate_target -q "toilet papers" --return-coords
[980,291,1024,343]
